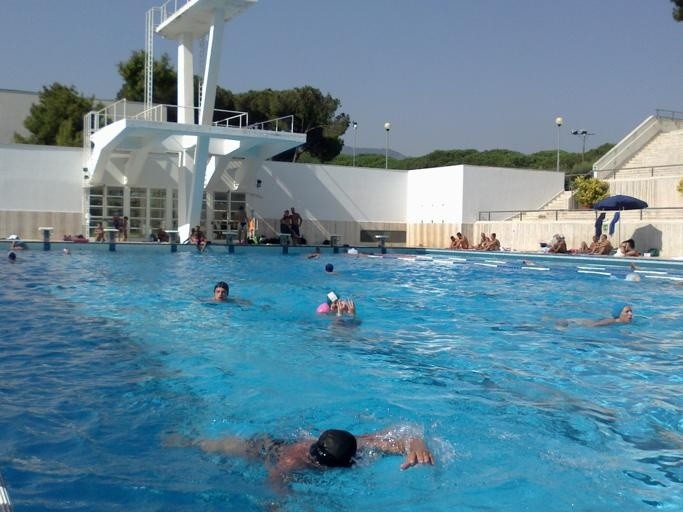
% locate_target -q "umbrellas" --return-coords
[592,195,648,246]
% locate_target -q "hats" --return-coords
[308,427,358,467]
[326,290,339,309]
[212,281,229,296]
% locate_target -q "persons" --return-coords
[336,299,355,317]
[281,207,320,259]
[548,234,640,257]
[237,205,249,244]
[11,241,27,250]
[543,301,633,329]
[325,263,337,275]
[9,252,16,261]
[206,282,243,303]
[94,213,129,243]
[193,428,436,503]
[157,225,210,251]
[449,232,500,252]
[317,292,339,313]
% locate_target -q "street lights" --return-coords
[350,120,359,168]
[382,122,393,170]
[554,115,564,176]
[570,127,597,167]
